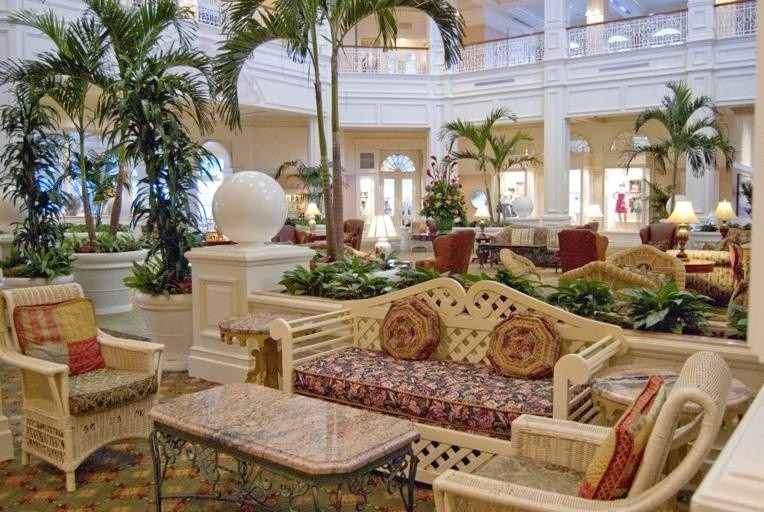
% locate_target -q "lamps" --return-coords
[584,203,604,222]
[304,202,321,233]
[712,200,738,245]
[664,201,701,262]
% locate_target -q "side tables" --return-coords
[218,311,319,390]
[590,363,749,485]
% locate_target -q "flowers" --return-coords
[416,153,466,218]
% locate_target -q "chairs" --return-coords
[433,348,733,512]
[0,282,165,492]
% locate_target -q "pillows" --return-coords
[577,374,666,500]
[486,313,562,380]
[378,295,441,361]
[13,298,105,376]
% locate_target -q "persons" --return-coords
[500,187,514,219]
[612,181,632,223]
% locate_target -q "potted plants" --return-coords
[436,105,544,244]
[209,0,467,351]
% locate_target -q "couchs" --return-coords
[269,277,629,486]
